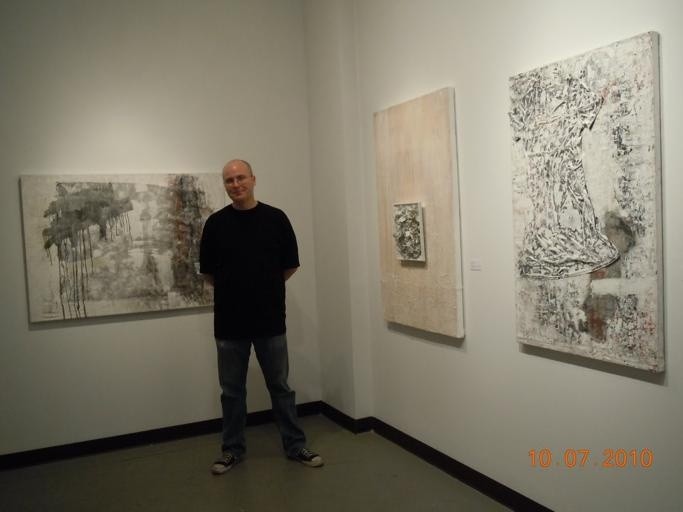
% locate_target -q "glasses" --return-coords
[223,175,249,185]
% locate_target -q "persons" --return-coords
[199,159,323,475]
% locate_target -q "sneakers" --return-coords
[295,448,324,467]
[211,452,239,475]
